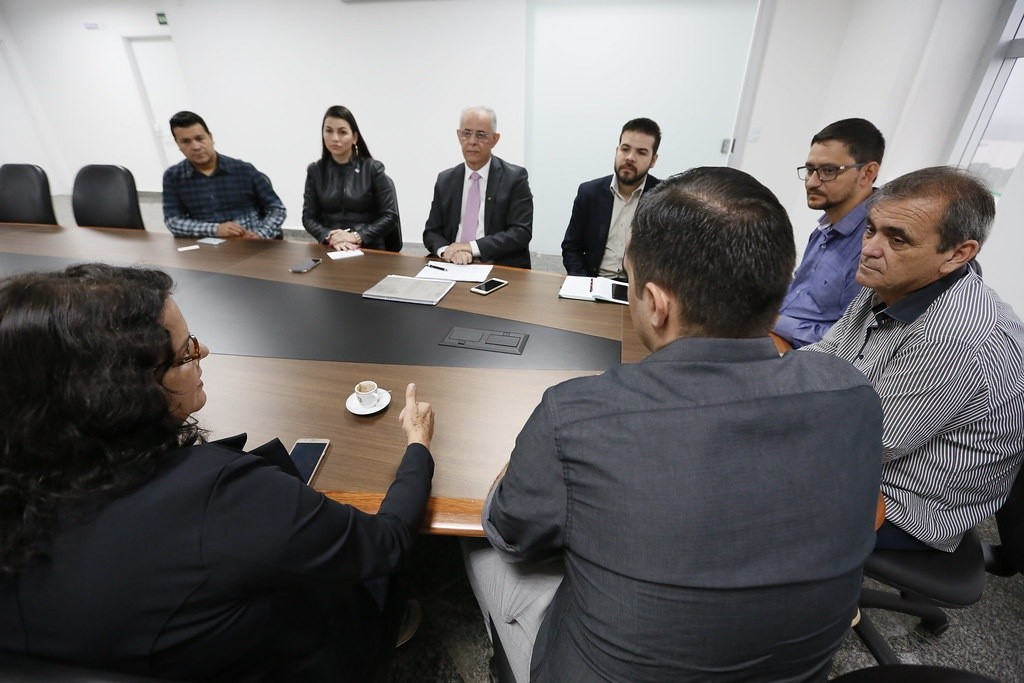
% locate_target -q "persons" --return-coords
[303,106,398,251]
[162,111,287,239]
[423,106,533,269]
[560,118,662,279]
[771,117,886,349]
[462,165,884,683]
[794,165,1024,554]
[0,261,434,683]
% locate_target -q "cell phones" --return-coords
[611,284,628,302]
[470,278,508,296]
[289,438,330,488]
[291,258,322,273]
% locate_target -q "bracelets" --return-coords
[354,232,362,245]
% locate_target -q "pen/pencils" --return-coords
[590,279,593,292]
[325,228,351,239]
[425,264,447,271]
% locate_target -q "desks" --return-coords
[0,224,885,545]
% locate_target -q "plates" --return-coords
[346,387,391,415]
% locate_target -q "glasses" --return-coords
[169,336,201,370]
[797,161,870,181]
[458,130,493,142]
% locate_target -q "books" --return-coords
[560,276,629,305]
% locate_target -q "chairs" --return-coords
[0,163,59,225]
[382,174,404,252]
[71,164,147,229]
[844,452,1024,664]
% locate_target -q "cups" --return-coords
[355,381,380,407]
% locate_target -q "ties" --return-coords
[459,170,480,244]
